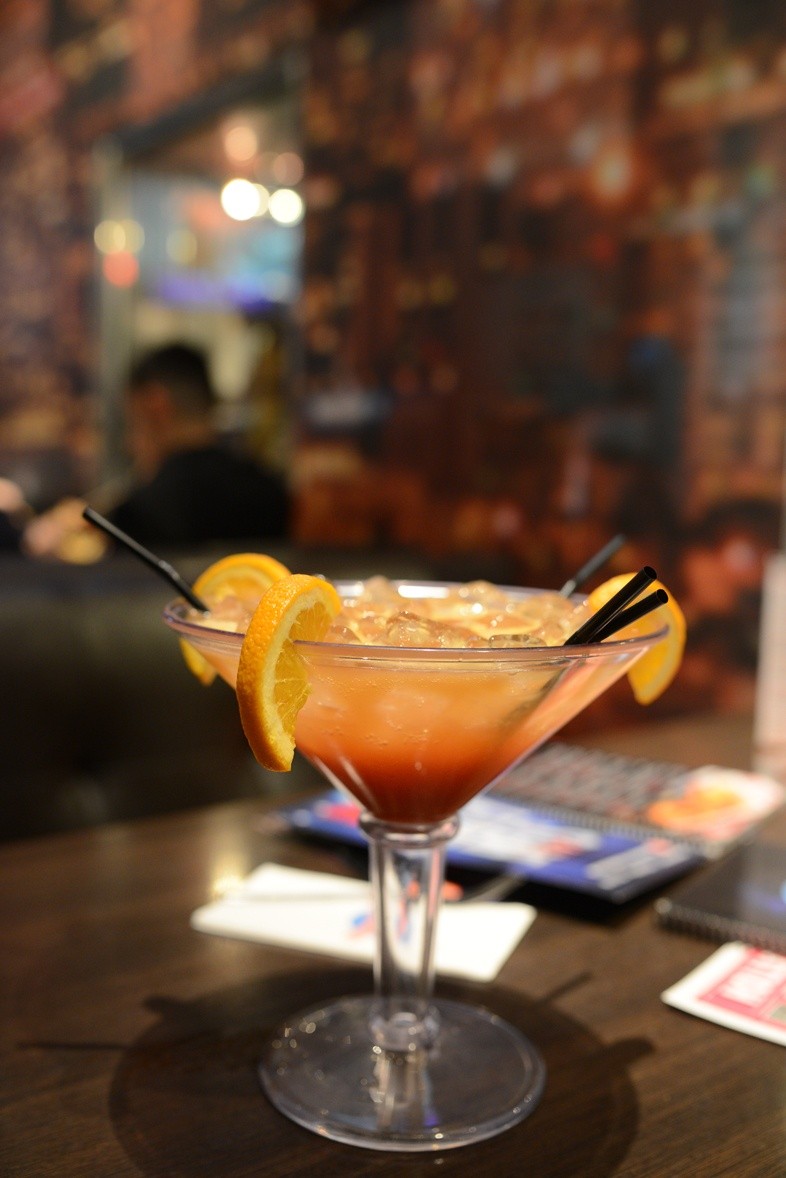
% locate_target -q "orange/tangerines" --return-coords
[585,572,685,705]
[179,552,342,772]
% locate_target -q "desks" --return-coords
[0,711,786,1178]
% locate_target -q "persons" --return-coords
[104,340,297,550]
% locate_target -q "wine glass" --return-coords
[159,577,673,1155]
